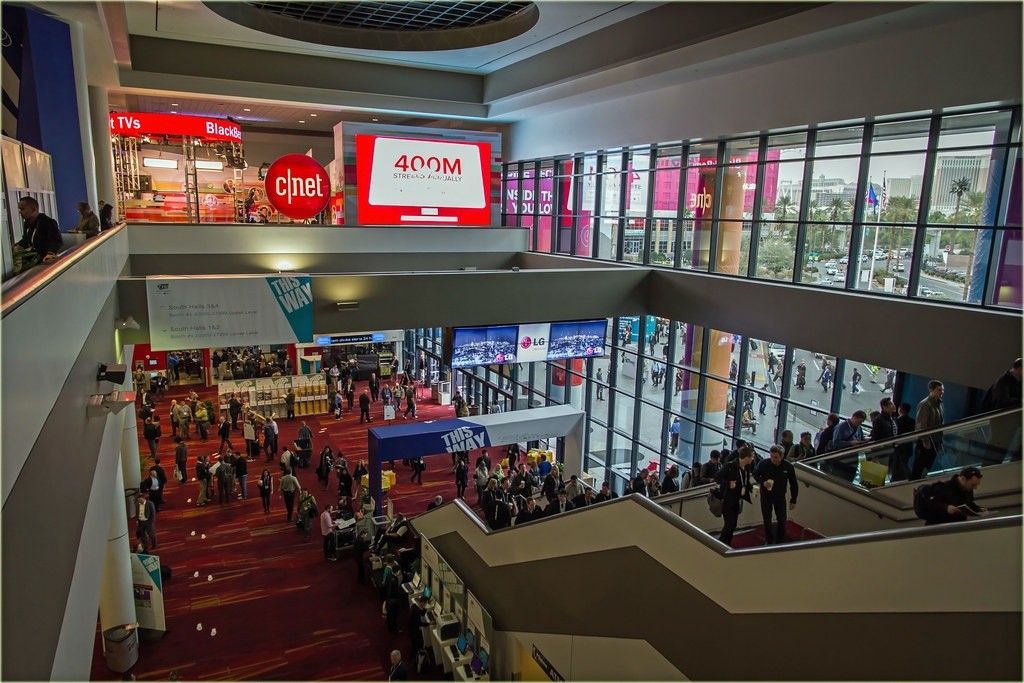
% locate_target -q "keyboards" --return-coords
[415,597,419,603]
[450,645,460,658]
[404,582,412,592]
[463,664,474,678]
[426,611,435,622]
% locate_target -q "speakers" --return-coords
[105,363,127,385]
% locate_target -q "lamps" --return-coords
[115,316,140,330]
[95,362,127,386]
[87,390,136,420]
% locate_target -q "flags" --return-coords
[865,178,886,214]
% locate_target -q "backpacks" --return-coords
[979,373,1012,421]
[912,480,948,520]
[290,451,300,468]
[531,467,541,487]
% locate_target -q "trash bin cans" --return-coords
[431,384,438,399]
[468,405,478,415]
[102,623,139,673]
[125,489,137,517]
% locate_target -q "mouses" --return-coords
[475,677,480,680]
[455,659,459,661]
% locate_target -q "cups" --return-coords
[509,503,513,509]
[767,479,774,491]
[753,485,760,498]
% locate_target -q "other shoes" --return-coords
[412,415,418,418]
[237,492,247,500]
[458,496,466,501]
[402,415,407,420]
[196,498,212,506]
[335,416,343,420]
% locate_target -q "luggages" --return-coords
[317,467,325,480]
[296,518,305,529]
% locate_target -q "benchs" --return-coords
[386,526,420,572]
[725,418,752,432]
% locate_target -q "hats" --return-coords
[479,461,485,468]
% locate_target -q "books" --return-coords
[957,503,999,516]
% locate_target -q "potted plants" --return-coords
[204,399,216,425]
[328,389,337,413]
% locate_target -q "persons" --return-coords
[595,317,686,454]
[452,448,729,530]
[68,202,99,239]
[715,427,823,545]
[225,180,235,193]
[451,390,502,417]
[133,415,279,554]
[133,345,296,438]
[330,355,427,423]
[14,196,62,261]
[354,512,433,682]
[506,363,523,392]
[729,334,806,435]
[278,420,422,560]
[817,358,1022,530]
[99,200,114,231]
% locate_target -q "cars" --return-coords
[833,272,845,283]
[822,277,833,288]
[826,255,848,268]
[899,284,947,300]
[875,248,913,261]
[768,341,796,364]
[926,262,972,277]
[858,254,869,263]
[826,265,838,275]
[892,263,905,273]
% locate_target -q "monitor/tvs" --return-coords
[546,319,608,362]
[423,586,432,600]
[470,652,483,674]
[451,325,519,368]
[433,601,443,617]
[412,571,422,589]
[457,632,467,655]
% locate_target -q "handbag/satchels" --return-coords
[860,456,889,489]
[201,421,212,430]
[501,458,509,469]
[419,460,427,471]
[335,407,340,415]
[708,487,725,517]
[825,440,837,456]
[174,464,184,480]
